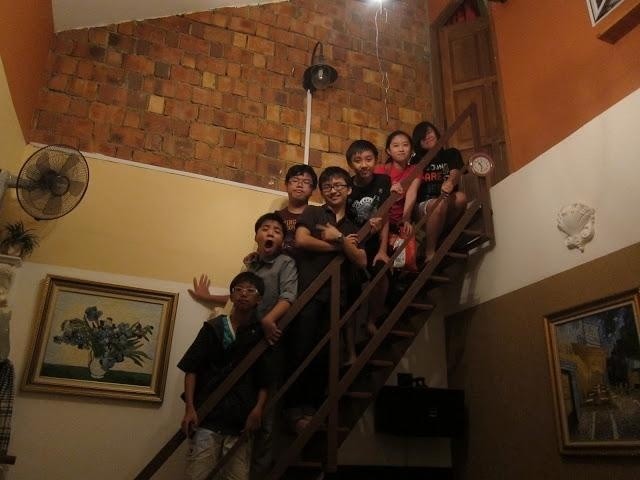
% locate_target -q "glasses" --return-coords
[289,177,313,186]
[233,286,260,295]
[319,183,348,190]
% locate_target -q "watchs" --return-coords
[337,233,344,245]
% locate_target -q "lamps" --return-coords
[303,41,337,95]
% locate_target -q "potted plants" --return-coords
[0,221,40,312]
[52,305,155,378]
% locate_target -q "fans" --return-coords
[1,143,89,221]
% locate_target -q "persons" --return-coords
[409,122,468,267]
[274,164,318,250]
[346,140,395,277]
[294,167,368,422]
[188,213,299,439]
[373,130,448,265]
[177,271,285,479]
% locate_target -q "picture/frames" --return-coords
[18,272,179,408]
[541,288,640,460]
